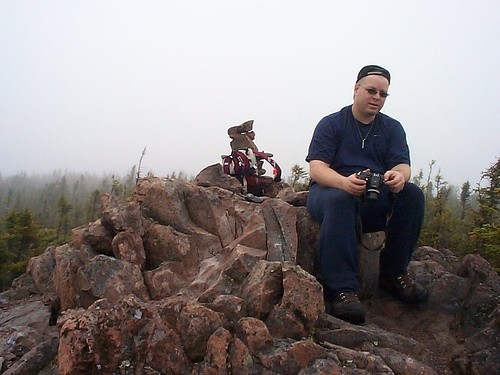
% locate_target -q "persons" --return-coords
[307,65,430,324]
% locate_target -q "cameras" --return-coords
[355,171,385,201]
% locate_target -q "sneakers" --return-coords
[378,271,430,305]
[330,290,365,324]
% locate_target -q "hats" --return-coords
[356,65,390,85]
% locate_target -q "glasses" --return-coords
[358,83,389,98]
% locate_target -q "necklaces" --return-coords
[355,116,375,149]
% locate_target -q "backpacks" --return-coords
[221,146,282,195]
